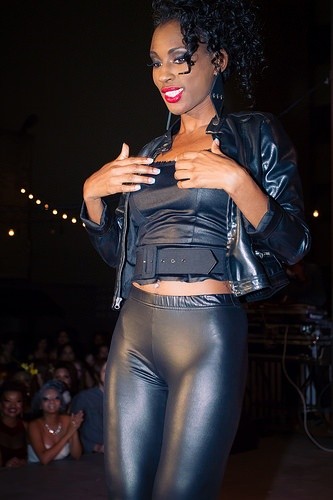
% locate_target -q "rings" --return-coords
[72,420,75,424]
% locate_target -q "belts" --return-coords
[133,245,228,280]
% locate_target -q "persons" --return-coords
[0,380,29,472]
[28,381,84,465]
[0,330,112,422]
[80,0,311,500]
[69,356,108,453]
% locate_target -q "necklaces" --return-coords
[45,422,63,435]
